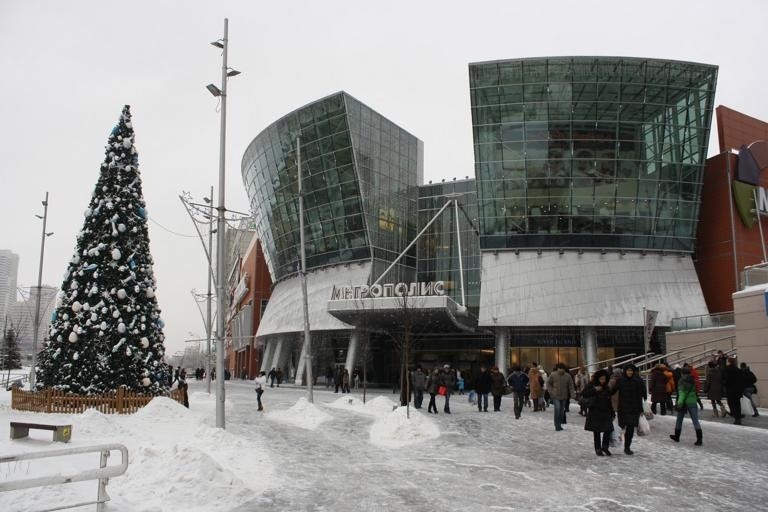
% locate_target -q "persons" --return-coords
[610,362,645,455]
[172,366,358,411]
[669,366,703,446]
[581,368,616,456]
[399,363,589,431]
[649,350,759,425]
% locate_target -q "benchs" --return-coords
[10,420,74,445]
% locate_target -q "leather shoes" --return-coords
[601,448,610,456]
[623,448,632,455]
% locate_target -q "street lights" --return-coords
[200,195,217,394]
[26,189,56,390]
[205,35,246,431]
[725,147,744,292]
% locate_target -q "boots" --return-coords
[712,409,717,418]
[720,405,727,417]
[669,428,681,442]
[694,429,702,446]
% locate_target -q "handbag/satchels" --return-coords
[538,376,544,387]
[675,404,686,413]
[438,385,448,396]
[579,382,596,408]
